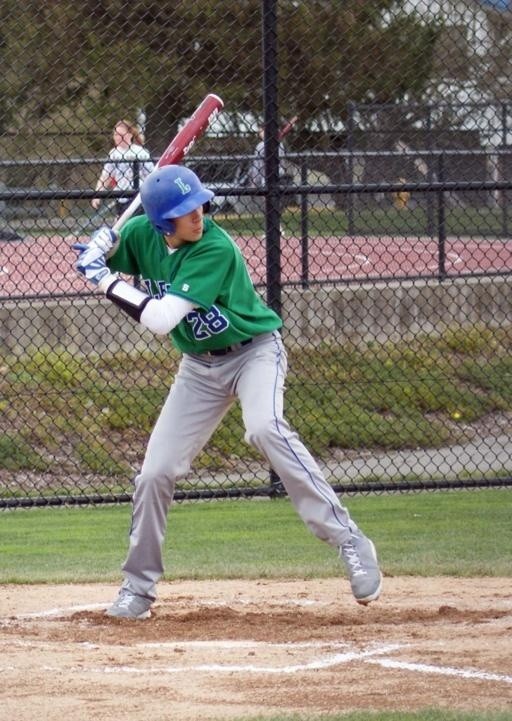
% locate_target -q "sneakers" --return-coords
[105,596,154,619]
[338,529,382,603]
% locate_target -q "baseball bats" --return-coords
[111,93,224,234]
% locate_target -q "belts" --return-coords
[208,337,253,356]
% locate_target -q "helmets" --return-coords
[141,164,216,237]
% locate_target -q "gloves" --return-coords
[91,228,121,261]
[73,244,110,285]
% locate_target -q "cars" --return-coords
[116,150,337,222]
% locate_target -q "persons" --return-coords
[74,164,382,620]
[91,120,155,220]
[251,122,287,239]
[177,116,215,214]
